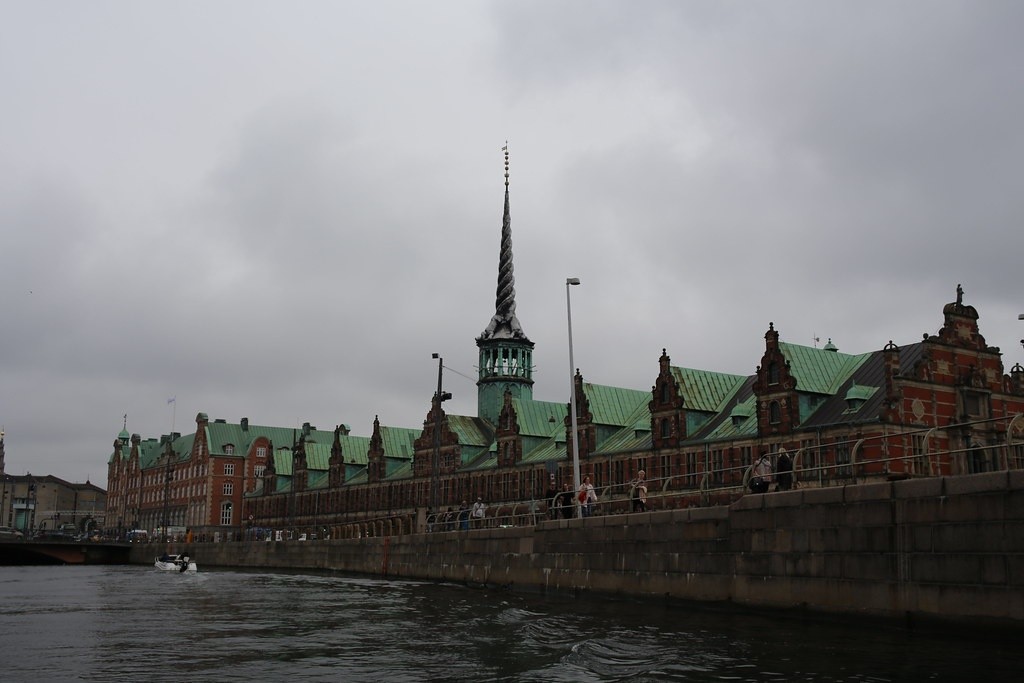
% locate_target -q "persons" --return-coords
[28,529,266,542]
[545,481,562,520]
[443,506,456,531]
[425,505,436,534]
[472,497,486,529]
[748,451,772,494]
[558,483,577,520]
[630,470,647,514]
[458,500,471,530]
[578,476,598,517]
[773,447,793,491]
[945,412,972,475]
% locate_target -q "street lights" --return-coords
[430,351,443,514]
[566,276,585,519]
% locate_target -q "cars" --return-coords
[0,525,23,541]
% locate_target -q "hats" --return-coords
[478,498,482,501]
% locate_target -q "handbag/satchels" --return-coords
[749,477,763,489]
[578,489,587,503]
[627,479,639,497]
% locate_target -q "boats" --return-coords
[154,554,198,574]
